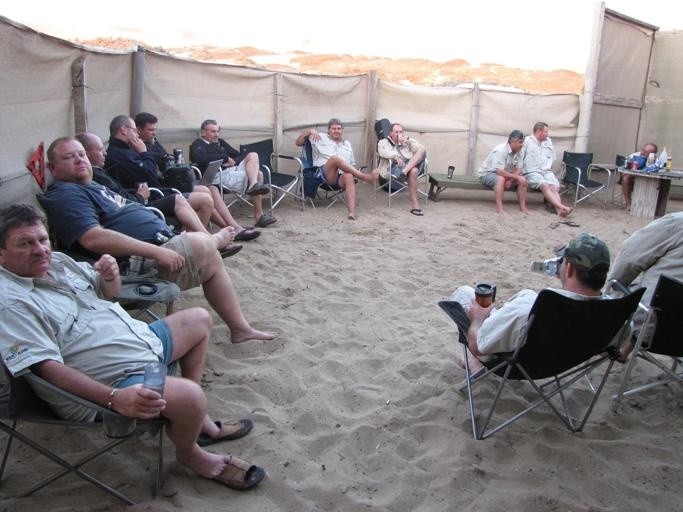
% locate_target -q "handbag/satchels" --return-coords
[164,168,193,192]
[300,176,319,198]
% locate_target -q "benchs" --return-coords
[429,169,566,203]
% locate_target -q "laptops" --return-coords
[195,159,223,186]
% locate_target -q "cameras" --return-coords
[531,255,564,277]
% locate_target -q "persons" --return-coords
[451,233,610,379]
[378,124,426,216]
[479,130,536,215]
[623,144,658,211]
[1,204,266,490]
[599,212,683,362]
[520,122,573,217]
[296,119,380,219]
[40,136,276,344]
[76,113,276,258]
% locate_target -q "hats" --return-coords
[564,234,610,273]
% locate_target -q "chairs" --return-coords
[437,274,649,441]
[238,140,307,217]
[294,139,365,214]
[560,150,611,209]
[0,140,272,506]
[371,139,427,211]
[592,272,682,417]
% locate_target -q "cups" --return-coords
[397,134,403,146]
[447,166,455,179]
[475,282,496,308]
[172,147,182,166]
[143,361,167,399]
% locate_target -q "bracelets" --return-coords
[108,389,118,409]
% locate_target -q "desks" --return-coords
[617,166,683,220]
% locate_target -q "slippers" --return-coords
[565,220,579,227]
[218,243,242,258]
[212,456,265,491]
[233,226,261,241]
[197,419,253,446]
[410,209,423,216]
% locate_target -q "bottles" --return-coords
[658,146,673,172]
[623,156,631,169]
[126,255,145,277]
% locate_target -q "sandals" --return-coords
[255,215,277,227]
[246,184,270,196]
